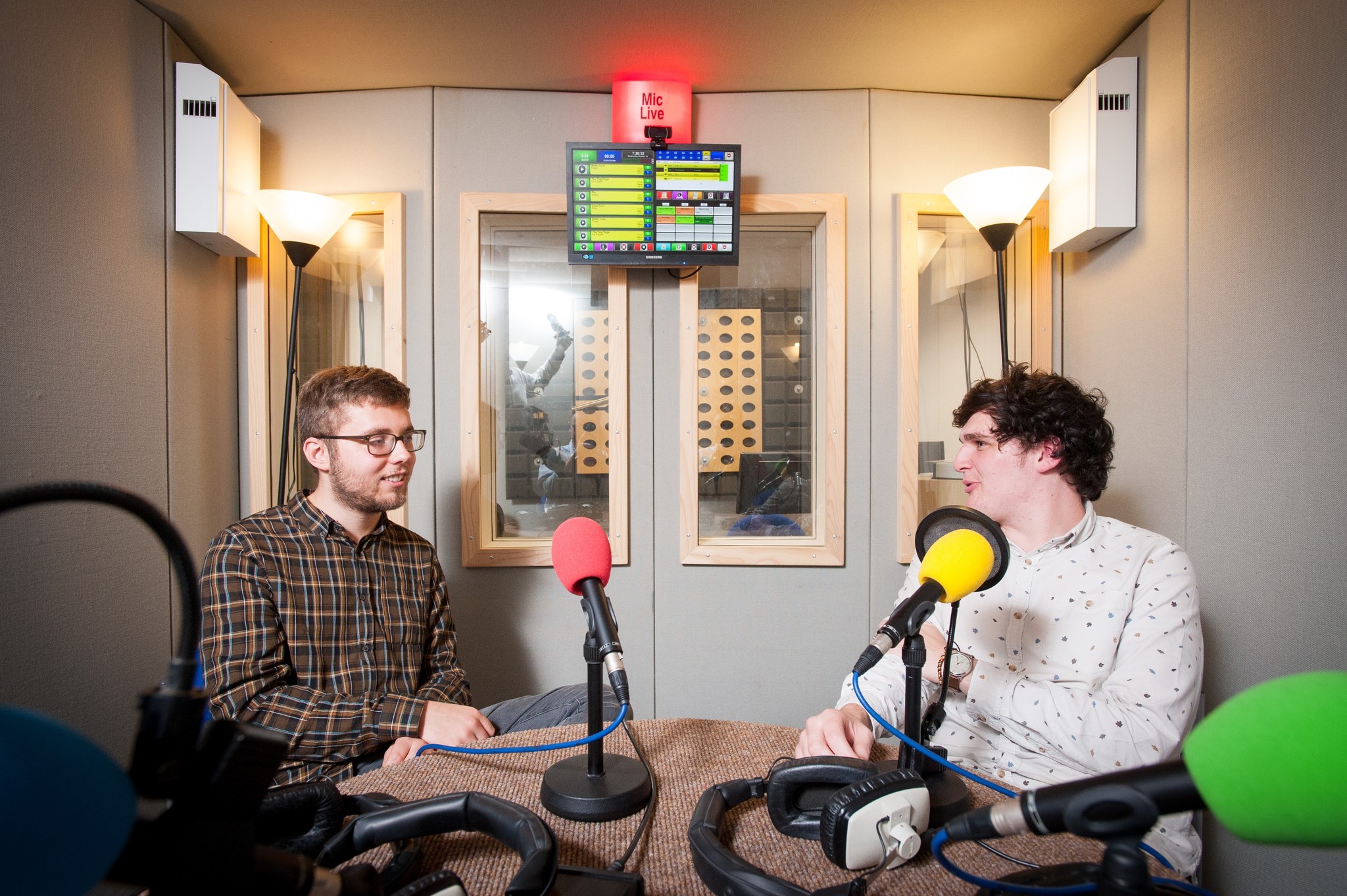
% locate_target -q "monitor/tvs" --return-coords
[735,451,796,515]
[565,141,742,267]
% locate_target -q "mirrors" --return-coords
[246,191,409,529]
[458,191,629,564]
[897,192,1052,564]
[678,191,846,567]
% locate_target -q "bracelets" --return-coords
[937,646,958,687]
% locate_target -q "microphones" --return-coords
[549,515,635,710]
[758,466,788,492]
[1,702,313,896]
[847,527,994,674]
[759,457,791,489]
[942,669,1345,848]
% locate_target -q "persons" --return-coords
[744,421,810,538]
[536,402,610,525]
[793,360,1207,890]
[197,362,635,790]
[506,328,574,505]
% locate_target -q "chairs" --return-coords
[0,701,137,896]
[918,441,945,475]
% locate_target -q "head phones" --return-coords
[688,748,932,896]
[249,776,557,895]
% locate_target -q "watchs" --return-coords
[552,352,565,360]
[947,651,975,693]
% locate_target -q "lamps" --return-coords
[322,218,384,369]
[918,230,948,275]
[944,167,1056,379]
[248,190,354,507]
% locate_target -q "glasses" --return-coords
[315,429,427,456]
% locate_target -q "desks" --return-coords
[322,718,1192,896]
